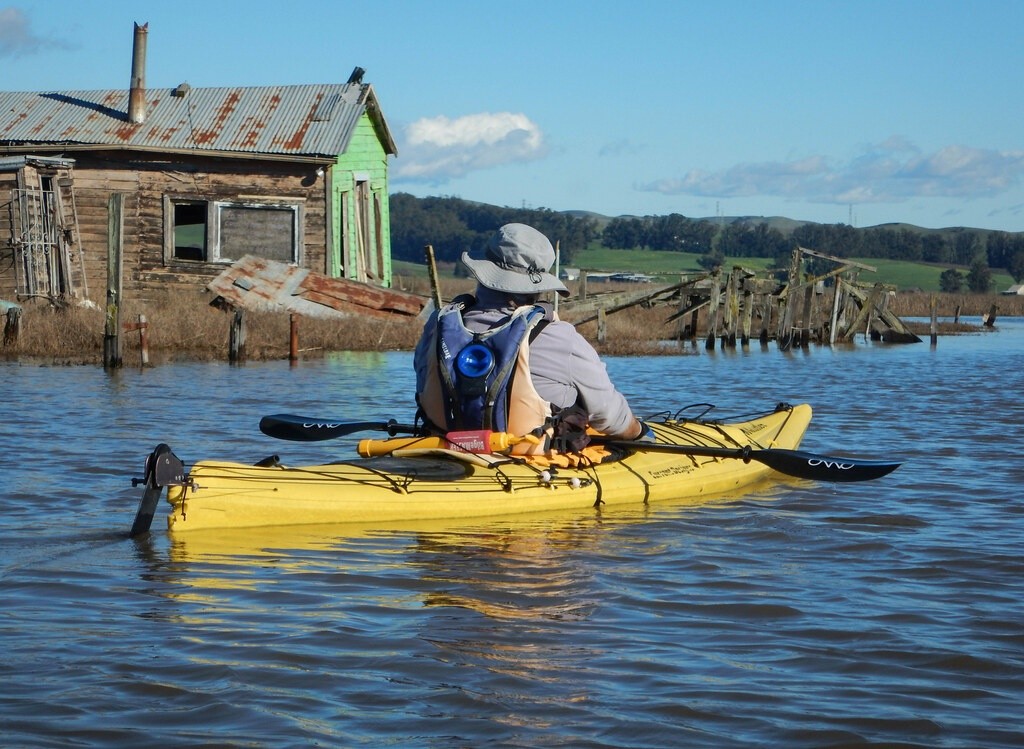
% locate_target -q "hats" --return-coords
[461,224,571,298]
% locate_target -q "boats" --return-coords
[127,399,812,542]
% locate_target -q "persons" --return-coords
[413,222,657,456]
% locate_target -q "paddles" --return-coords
[259,412,906,484]
